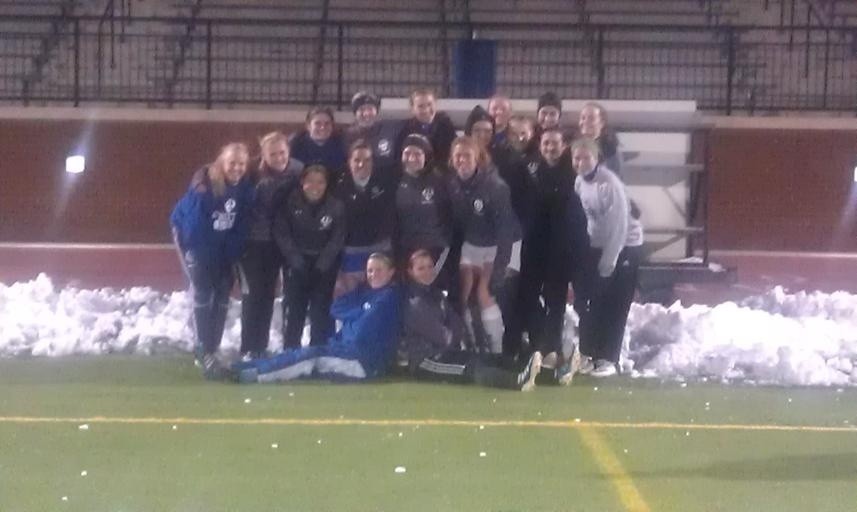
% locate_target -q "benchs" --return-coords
[0,1,857,114]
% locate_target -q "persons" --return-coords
[167,84,643,392]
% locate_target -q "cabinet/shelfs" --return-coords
[352,106,714,273]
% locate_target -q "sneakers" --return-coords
[193,351,224,383]
[517,345,619,393]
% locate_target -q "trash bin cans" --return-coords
[451,37,497,100]
